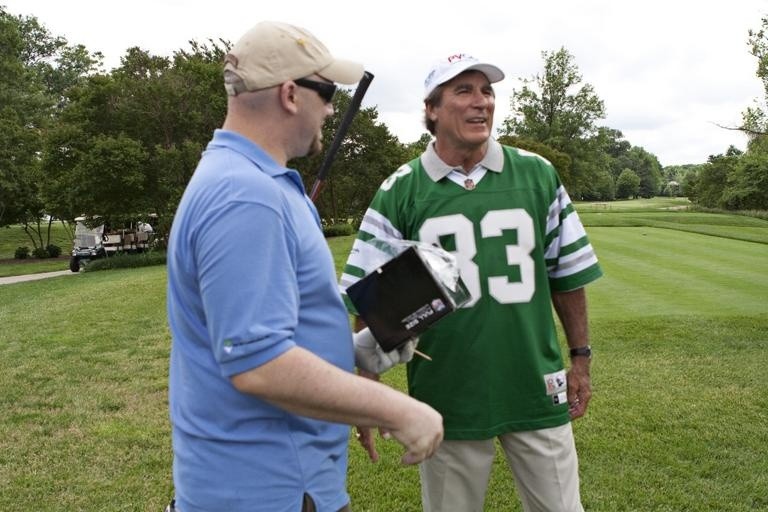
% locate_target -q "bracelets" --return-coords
[566,344,593,360]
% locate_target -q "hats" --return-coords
[423,52,505,104]
[222,19,365,96]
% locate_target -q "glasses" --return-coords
[292,78,341,107]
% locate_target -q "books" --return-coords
[345,245,456,354]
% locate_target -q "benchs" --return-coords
[103,233,149,251]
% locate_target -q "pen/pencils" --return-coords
[414,349,433,362]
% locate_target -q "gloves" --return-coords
[352,326,421,378]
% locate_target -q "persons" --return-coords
[137,220,153,233]
[165,21,446,511]
[335,49,605,511]
